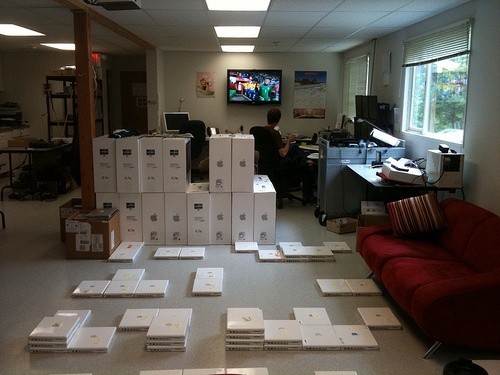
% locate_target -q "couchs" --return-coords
[356,197,500,359]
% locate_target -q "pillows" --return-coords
[387,191,447,239]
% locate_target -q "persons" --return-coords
[265,108,317,203]
[230,71,280,102]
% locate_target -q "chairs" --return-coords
[250,126,306,209]
[179,120,205,182]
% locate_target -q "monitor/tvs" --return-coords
[163,112,190,132]
[227,69,282,106]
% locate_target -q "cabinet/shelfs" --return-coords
[46,76,105,140]
[0,128,30,175]
[314,137,406,226]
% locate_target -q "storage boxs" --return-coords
[59,134,277,259]
[8,138,36,147]
[327,217,356,234]
[28,241,402,375]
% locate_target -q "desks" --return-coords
[0,142,72,201]
[346,163,466,202]
[299,144,319,204]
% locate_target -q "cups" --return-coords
[301,143,306,147]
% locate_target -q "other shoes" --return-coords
[305,196,317,203]
